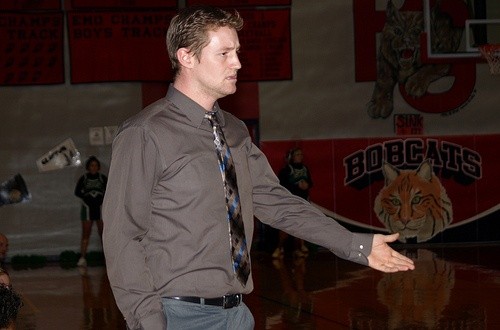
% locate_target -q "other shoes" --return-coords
[76,259,87,267]
[295,245,309,258]
[273,248,284,258]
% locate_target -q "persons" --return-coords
[101,8,415,330]
[0,234,24,330]
[280,146,312,200]
[76,157,110,266]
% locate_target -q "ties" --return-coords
[204,111,251,287]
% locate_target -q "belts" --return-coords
[162,295,242,309]
